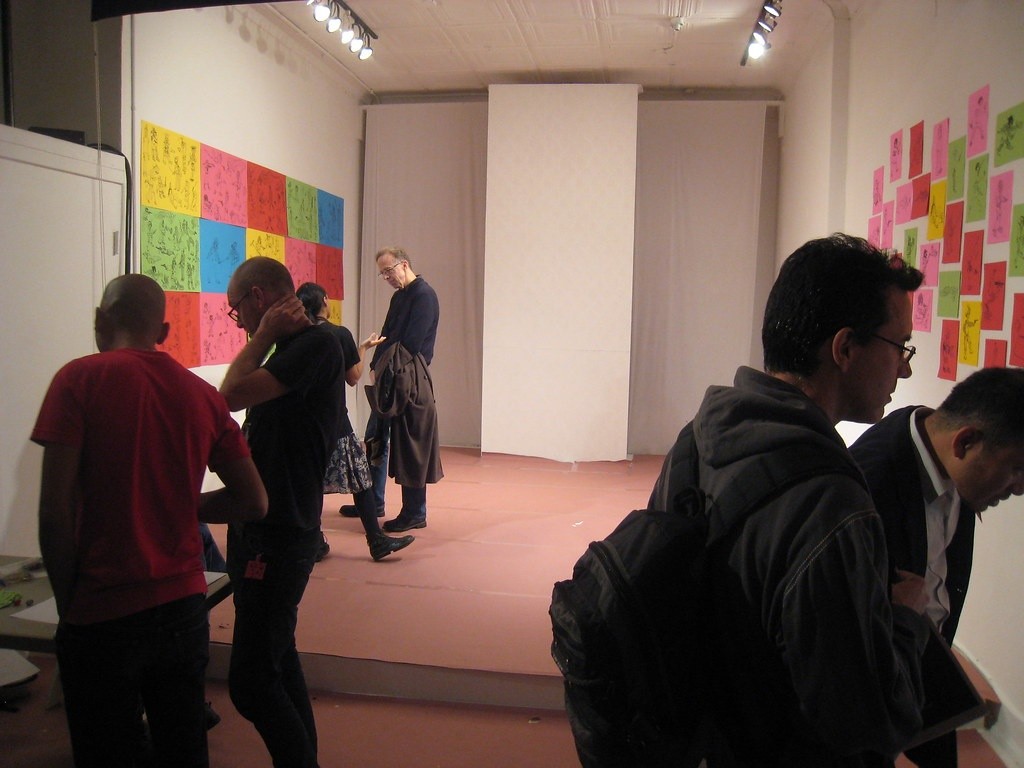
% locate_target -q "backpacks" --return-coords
[549,421,871,768]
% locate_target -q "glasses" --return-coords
[228,292,249,321]
[378,262,402,279]
[867,328,916,363]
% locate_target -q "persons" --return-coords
[30,273,269,768]
[296,282,415,563]
[218,255,346,768]
[339,245,439,534]
[847,367,1024,768]
[644,233,929,768]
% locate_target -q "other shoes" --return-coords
[206,700,221,731]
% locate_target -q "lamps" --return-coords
[740,0,783,66]
[304,0,378,60]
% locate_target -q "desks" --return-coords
[0,554,232,712]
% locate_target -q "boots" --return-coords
[315,531,330,563]
[365,529,415,561]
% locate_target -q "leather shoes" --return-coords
[382,513,427,531]
[339,502,386,518]
